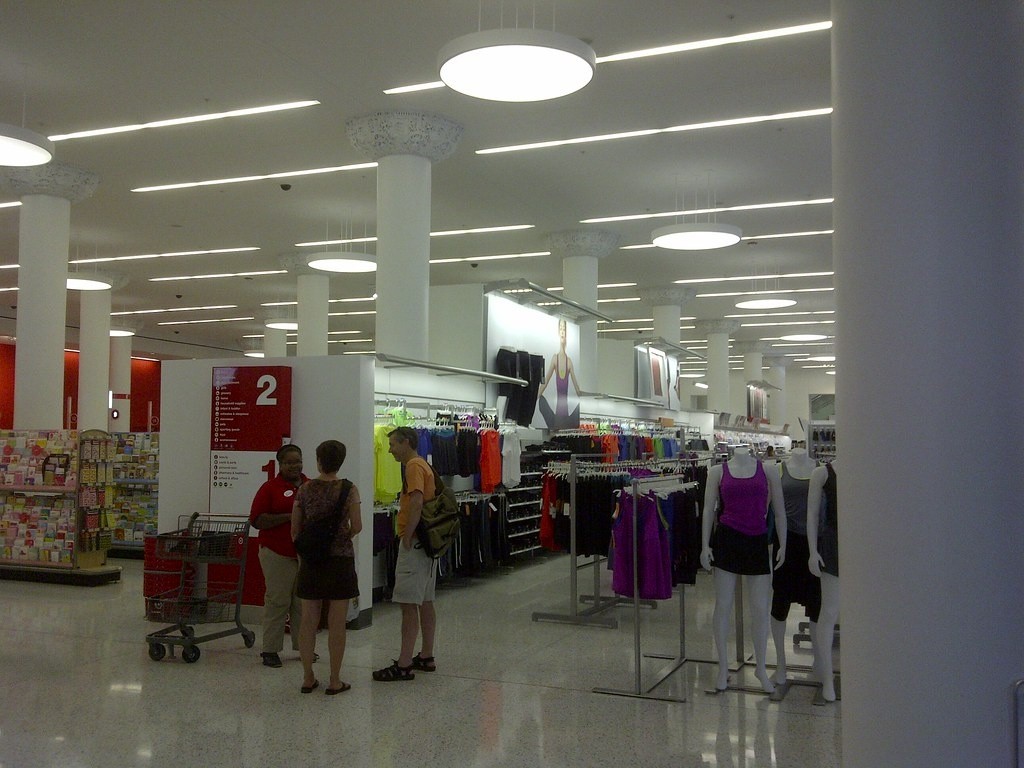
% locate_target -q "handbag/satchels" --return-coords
[294,478,354,564]
[401,460,460,558]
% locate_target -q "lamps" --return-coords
[651,173,743,250]
[437,1,597,103]
[734,265,798,310]
[244,338,264,359]
[67,240,113,291]
[264,288,298,330]
[305,219,376,273]
[110,303,136,336]
[0,94,55,167]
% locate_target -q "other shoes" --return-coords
[263,652,282,668]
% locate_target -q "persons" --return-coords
[770,447,827,685]
[291,439,362,696]
[537,318,581,432]
[372,426,443,682]
[805,458,844,701]
[699,447,788,694]
[249,444,320,668]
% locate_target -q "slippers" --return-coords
[300,679,319,693]
[325,681,351,695]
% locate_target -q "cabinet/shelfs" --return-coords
[0,428,122,587]
[494,472,544,558]
[109,432,160,560]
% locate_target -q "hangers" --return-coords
[613,476,700,500]
[555,417,676,440]
[459,420,516,436]
[541,456,708,480]
[405,416,454,430]
[455,492,505,504]
[375,414,396,427]
[437,403,481,420]
[374,500,400,516]
[384,398,406,412]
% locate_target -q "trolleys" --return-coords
[140,513,254,662]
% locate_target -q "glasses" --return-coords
[282,460,302,467]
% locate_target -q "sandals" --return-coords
[373,658,415,681]
[412,652,436,672]
